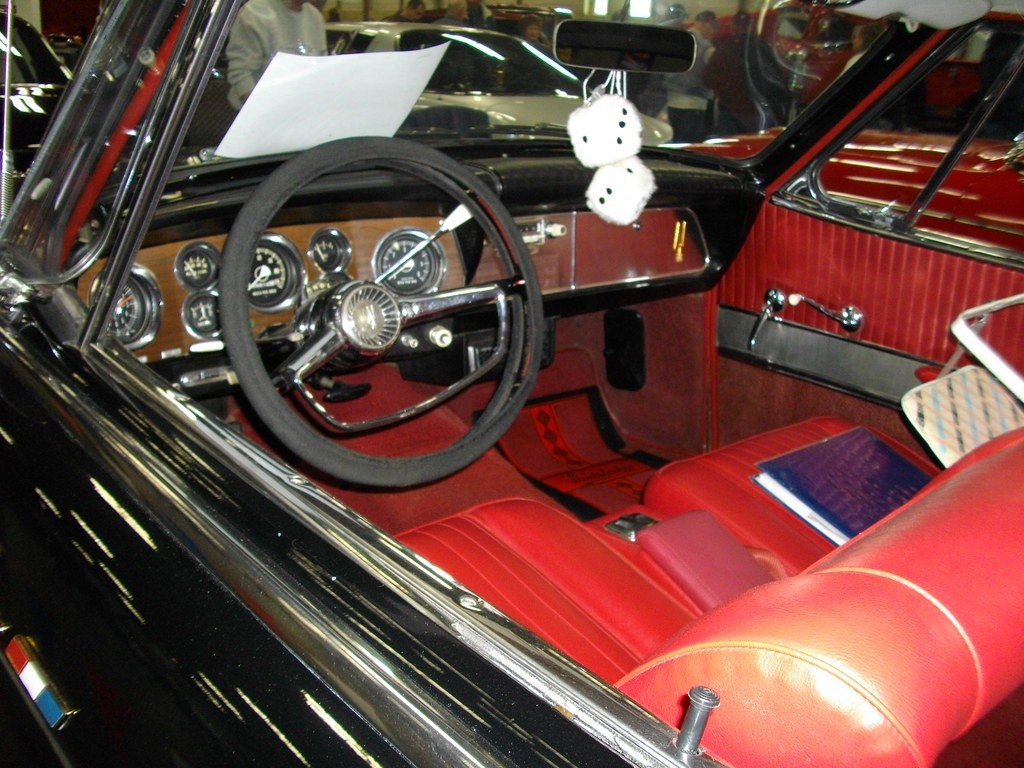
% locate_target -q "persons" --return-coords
[225,1,925,156]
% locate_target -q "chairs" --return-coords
[393,415,1024,768]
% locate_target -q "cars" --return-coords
[653,126,1024,257]
[319,18,672,151]
[0,13,76,214]
[0,0,1024,767]
[664,1,1024,132]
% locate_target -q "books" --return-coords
[750,426,932,547]
